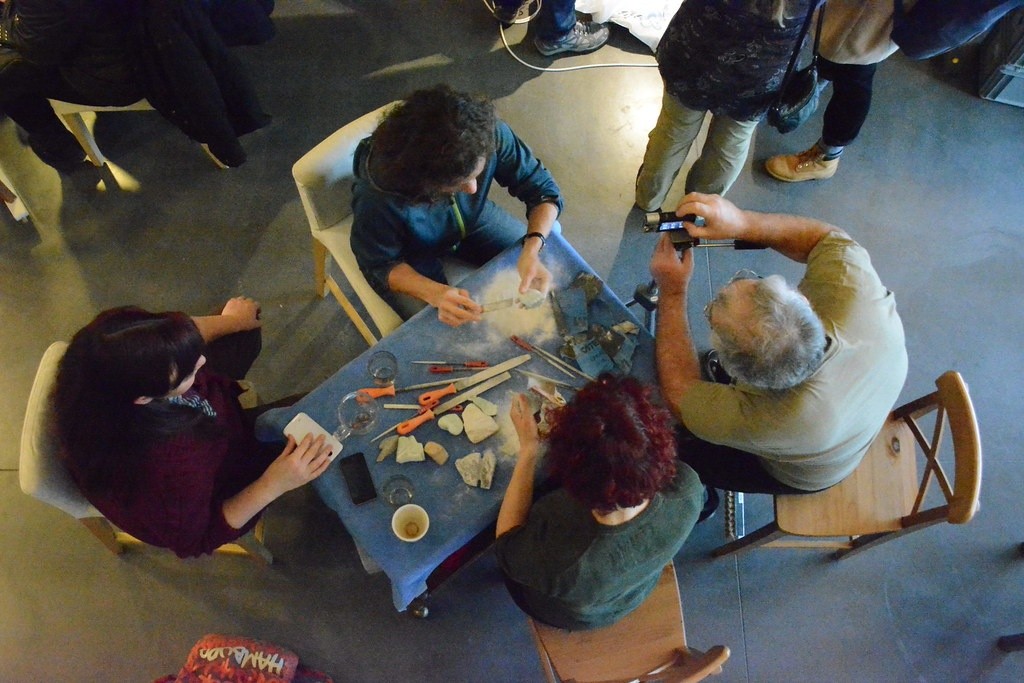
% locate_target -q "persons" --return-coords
[493,0,611,56]
[634,0,827,219]
[47,295,335,558]
[14,0,276,169]
[349,82,565,325]
[649,192,910,525]
[763,0,923,182]
[494,370,706,632]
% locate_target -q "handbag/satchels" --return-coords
[767,1,828,134]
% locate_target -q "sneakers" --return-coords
[764,143,840,183]
[533,19,611,56]
[493,0,536,25]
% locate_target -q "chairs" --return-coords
[18,341,275,567]
[292,101,483,352]
[43,0,238,169]
[710,372,984,563]
[154,634,334,683]
[529,559,732,683]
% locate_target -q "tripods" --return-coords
[624,229,771,338]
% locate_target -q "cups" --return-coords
[331,390,380,441]
[382,474,415,507]
[367,351,399,386]
[392,503,429,542]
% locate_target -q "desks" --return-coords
[264,228,663,611]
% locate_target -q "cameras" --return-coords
[642,211,705,233]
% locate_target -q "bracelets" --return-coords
[521,232,546,252]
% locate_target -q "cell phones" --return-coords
[282,412,343,463]
[337,452,377,506]
